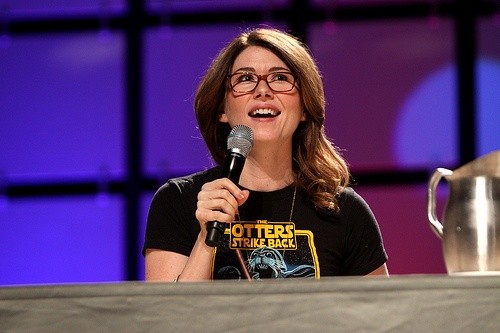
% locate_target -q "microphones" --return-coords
[205,124,254,247]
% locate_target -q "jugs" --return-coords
[426,149,500,276]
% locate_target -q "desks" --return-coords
[0,277,500,333]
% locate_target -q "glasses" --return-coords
[224,72,302,92]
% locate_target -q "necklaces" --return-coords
[237,185,296,278]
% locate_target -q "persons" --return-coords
[142,28,388,283]
